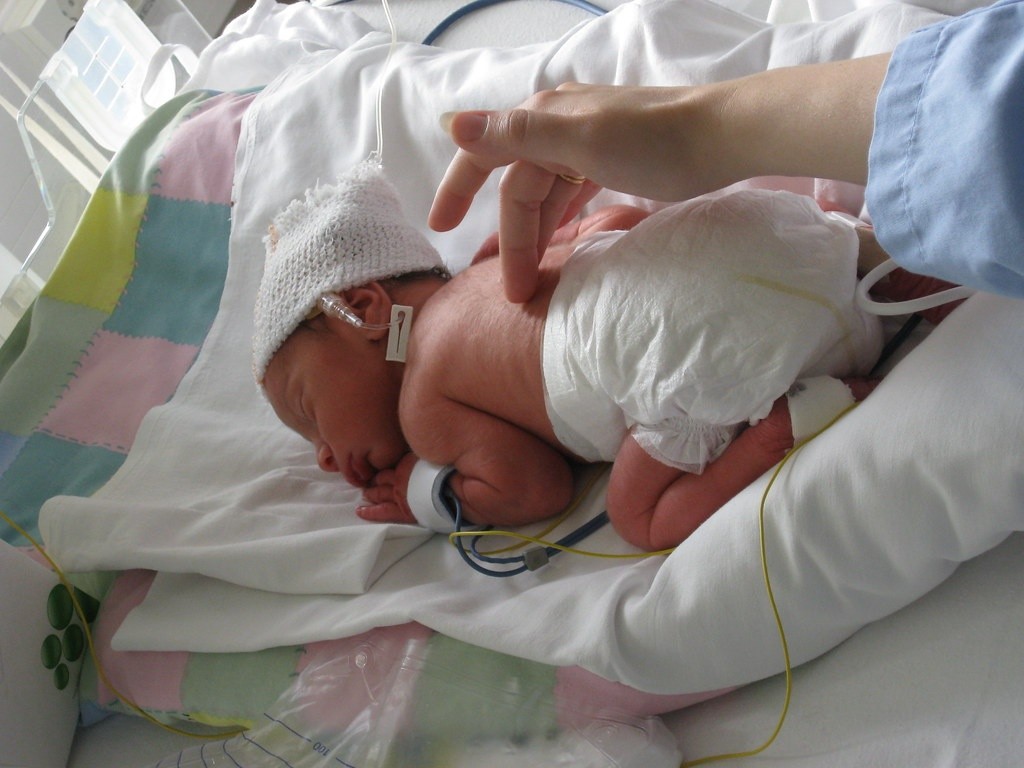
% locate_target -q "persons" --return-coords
[426,1,1024,303]
[249,164,970,552]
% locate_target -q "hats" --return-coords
[252,161,448,396]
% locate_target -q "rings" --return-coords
[560,173,587,184]
